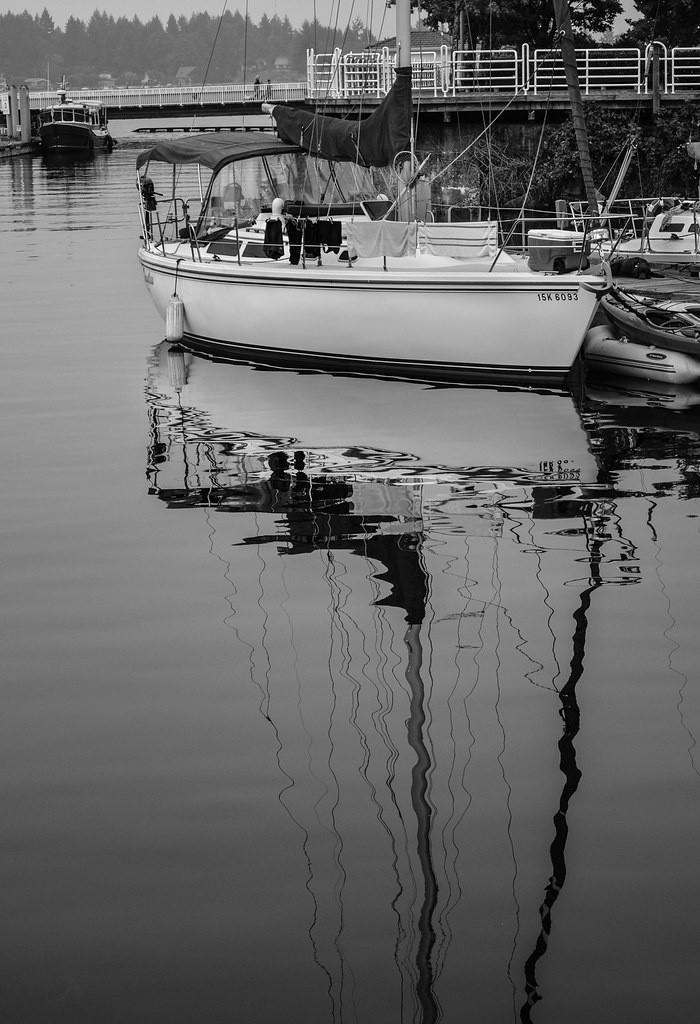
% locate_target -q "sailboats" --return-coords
[130,0,642,393]
[145,337,598,1024]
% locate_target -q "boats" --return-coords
[583,369,700,418]
[38,72,118,161]
[568,194,700,385]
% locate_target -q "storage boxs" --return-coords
[527,229,589,273]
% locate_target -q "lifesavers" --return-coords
[104,136,113,149]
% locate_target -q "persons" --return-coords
[254,75,272,100]
